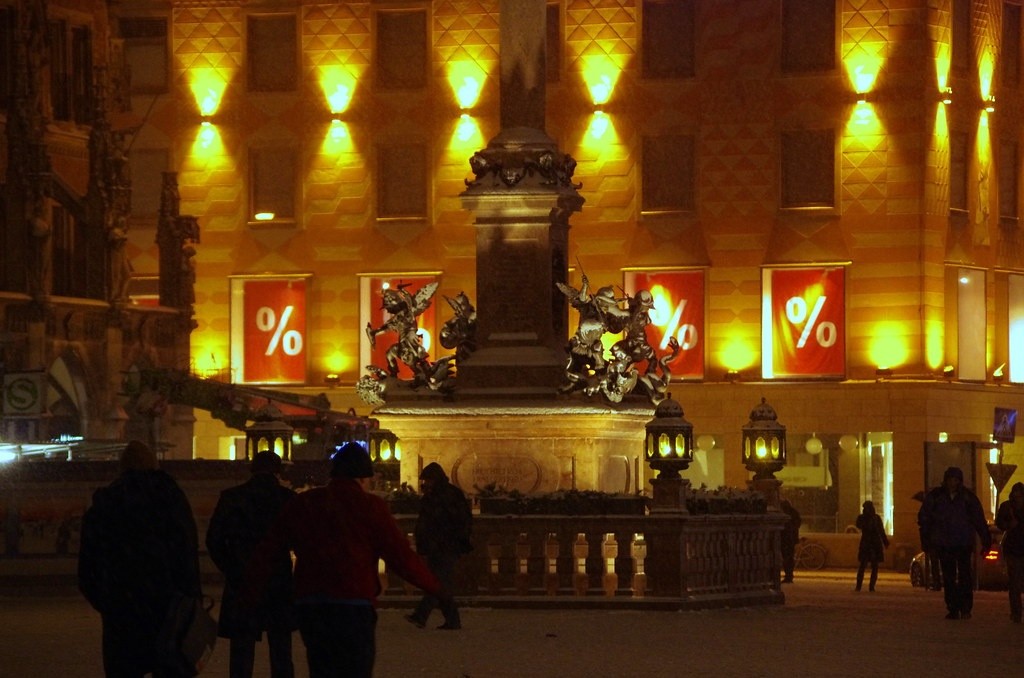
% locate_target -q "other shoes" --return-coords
[404,614,426,628]
[945,611,959,619]
[436,620,461,630]
[961,610,972,619]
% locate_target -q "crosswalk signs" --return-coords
[993,407,1016,443]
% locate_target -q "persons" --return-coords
[781,499,802,584]
[370,286,431,388]
[994,482,1024,624]
[610,289,659,377]
[240,442,441,678]
[439,294,477,356]
[566,274,626,372]
[403,461,473,631]
[204,450,300,678]
[854,500,889,592]
[917,466,992,620]
[77,439,206,678]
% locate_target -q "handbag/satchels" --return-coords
[175,595,219,678]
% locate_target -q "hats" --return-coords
[119,439,158,474]
[328,442,375,480]
